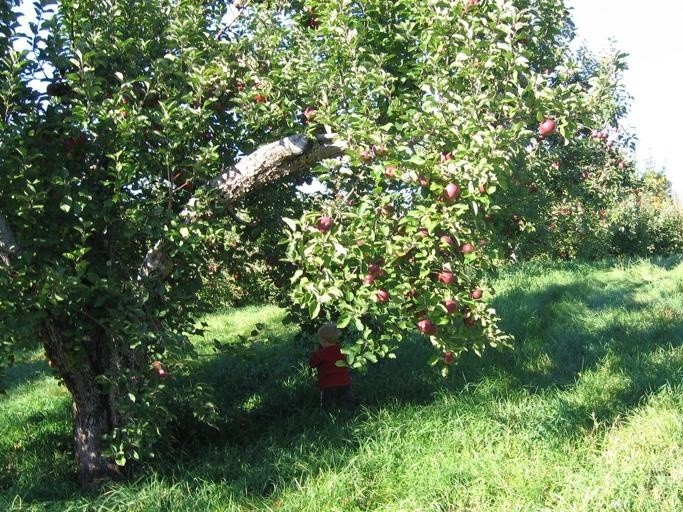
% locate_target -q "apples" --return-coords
[63,0,623,379]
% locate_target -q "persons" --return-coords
[307,324,354,411]
